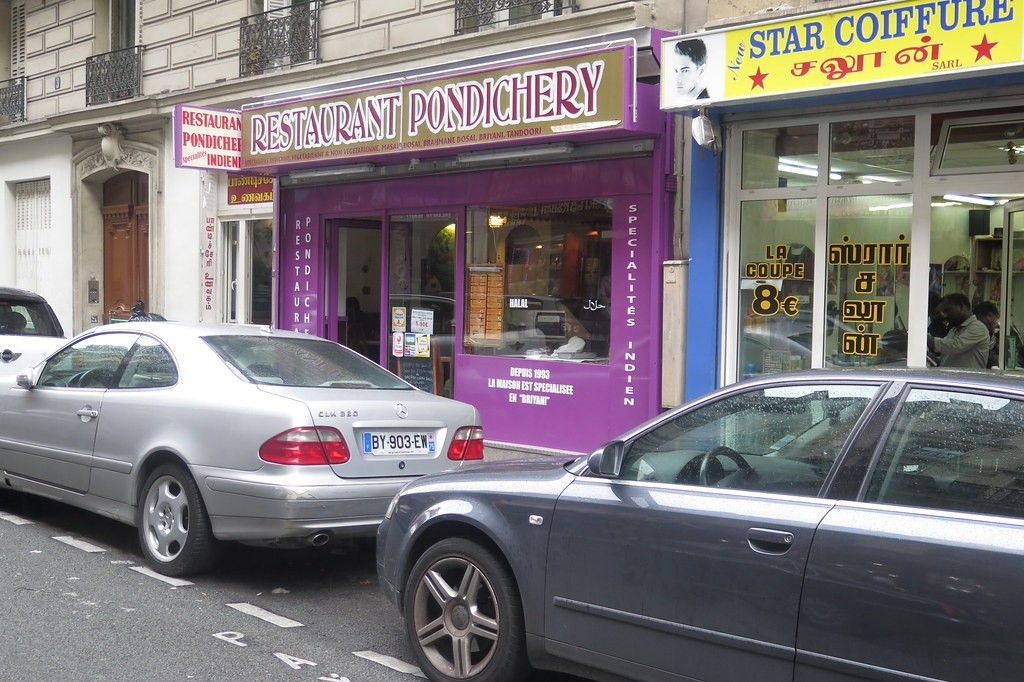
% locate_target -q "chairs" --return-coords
[246,364,274,377]
[346,296,372,340]
[0,312,27,334]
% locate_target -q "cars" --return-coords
[0,286,69,376]
[0,321,486,579]
[736,309,908,384]
[375,290,610,360]
[374,367,1024,682]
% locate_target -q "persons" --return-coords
[670,39,710,99]
[552,314,593,355]
[927,291,990,367]
[346,297,370,336]
[973,301,1024,369]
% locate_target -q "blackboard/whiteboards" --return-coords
[397,339,441,395]
